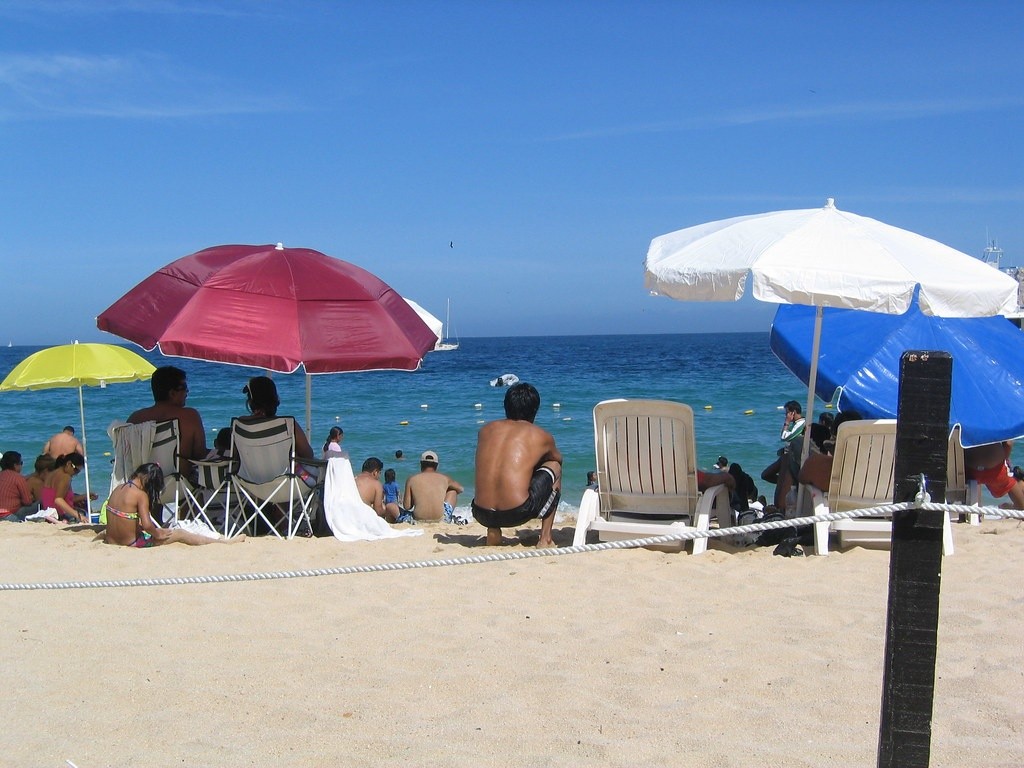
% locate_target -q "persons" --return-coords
[471,382,563,549]
[495,378,503,387]
[586,471,599,489]
[230,376,316,537]
[106,463,246,547]
[964,440,1024,510]
[322,426,464,523]
[0,426,98,523]
[126,366,208,522]
[696,400,847,516]
[203,427,233,462]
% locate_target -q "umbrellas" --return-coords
[0,340,157,523]
[770,283,1024,449]
[94,242,443,445]
[644,197,1019,519]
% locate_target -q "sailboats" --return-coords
[436,299,461,350]
[8,341,12,347]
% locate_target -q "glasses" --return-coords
[17,460,24,466]
[72,464,81,474]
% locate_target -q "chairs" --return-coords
[573,397,735,556]
[800,418,955,558]
[181,453,251,534]
[215,415,328,542]
[106,418,212,530]
[946,428,981,526]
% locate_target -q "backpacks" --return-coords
[735,509,760,540]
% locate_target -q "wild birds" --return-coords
[448,240,455,249]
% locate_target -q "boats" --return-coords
[980,239,1011,276]
[489,374,519,387]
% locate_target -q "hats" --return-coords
[420,450,439,464]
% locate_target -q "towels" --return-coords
[98,420,157,526]
[168,518,221,543]
[322,450,426,541]
